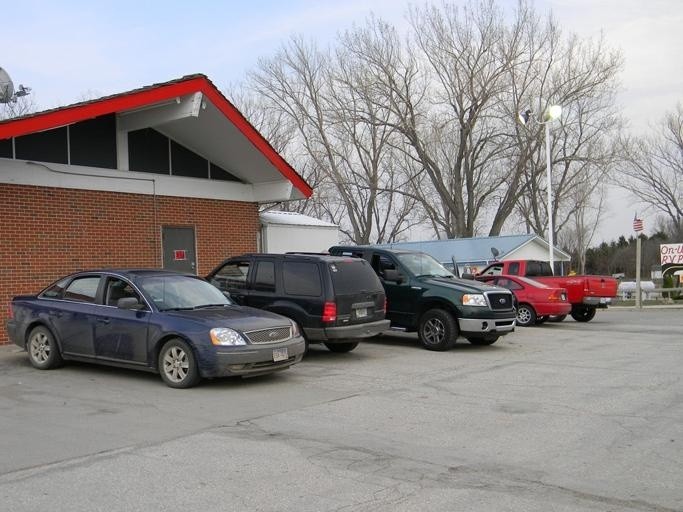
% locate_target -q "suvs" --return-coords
[204,249,391,356]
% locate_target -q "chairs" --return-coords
[108,285,126,306]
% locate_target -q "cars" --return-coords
[473,273,572,327]
[327,245,518,352]
[4,263,306,389]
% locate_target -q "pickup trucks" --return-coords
[477,258,619,322]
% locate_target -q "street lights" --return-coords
[519,102,564,275]
